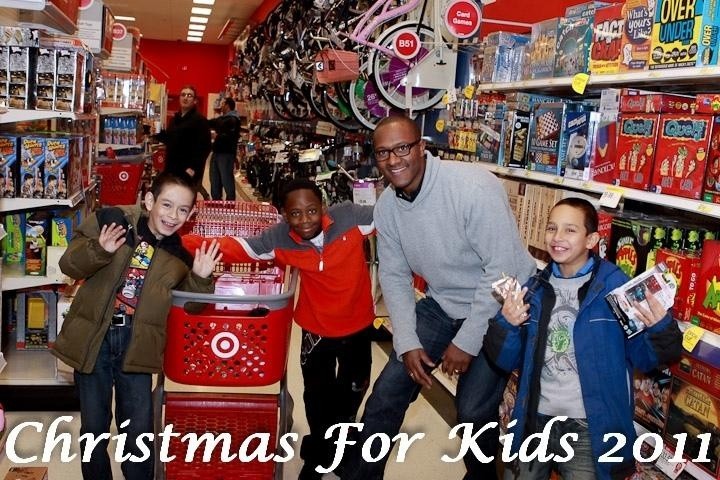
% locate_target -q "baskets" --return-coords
[314,48,359,84]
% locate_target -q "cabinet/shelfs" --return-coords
[1,22,147,385]
[382,63,720,480]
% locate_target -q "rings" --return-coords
[408,372,412,376]
[455,368,461,372]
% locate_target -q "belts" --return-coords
[111,315,126,327]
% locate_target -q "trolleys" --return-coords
[152,199,299,478]
[92,141,159,209]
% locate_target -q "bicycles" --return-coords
[237,122,384,215]
[224,0,482,135]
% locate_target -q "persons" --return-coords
[325,115,539,480]
[49,169,223,480]
[484,198,683,480]
[152,88,212,202]
[208,97,241,209]
[177,177,375,480]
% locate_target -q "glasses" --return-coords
[374,138,421,162]
[182,93,194,97]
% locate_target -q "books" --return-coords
[501,176,601,270]
